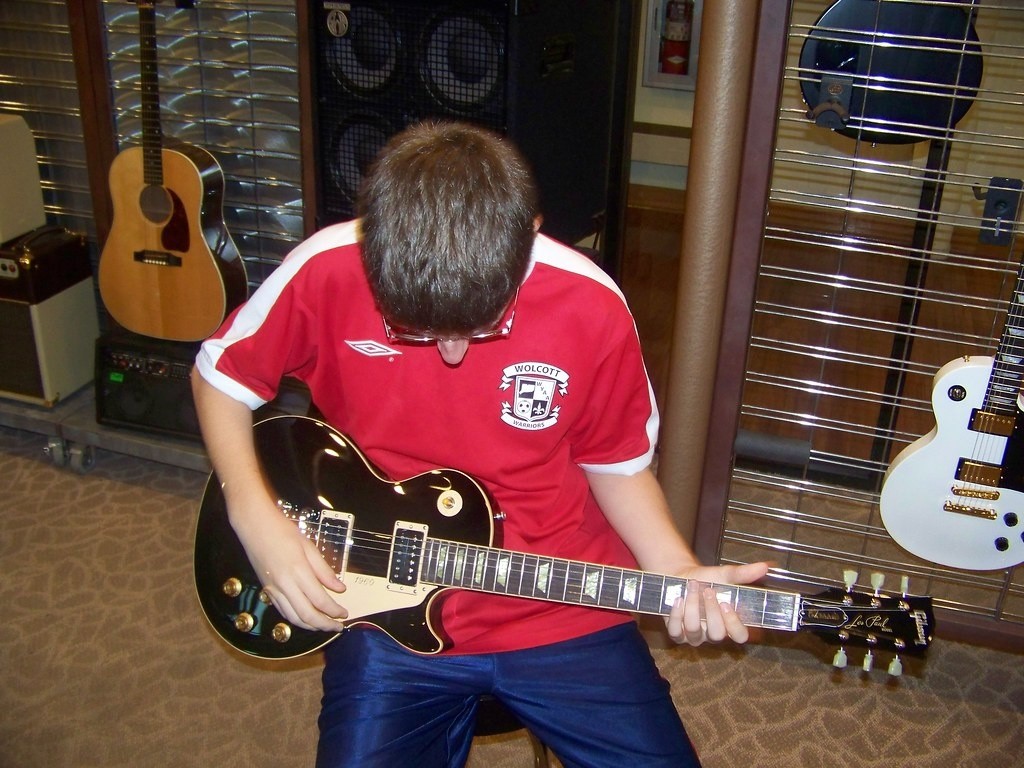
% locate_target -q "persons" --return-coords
[191,121,768,768]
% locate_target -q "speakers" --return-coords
[94,338,268,441]
[295,0,643,295]
[0,225,102,408]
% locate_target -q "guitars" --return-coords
[96,0,250,344]
[878,249,1024,572]
[192,413,935,678]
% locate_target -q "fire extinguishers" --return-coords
[658,0,694,75]
